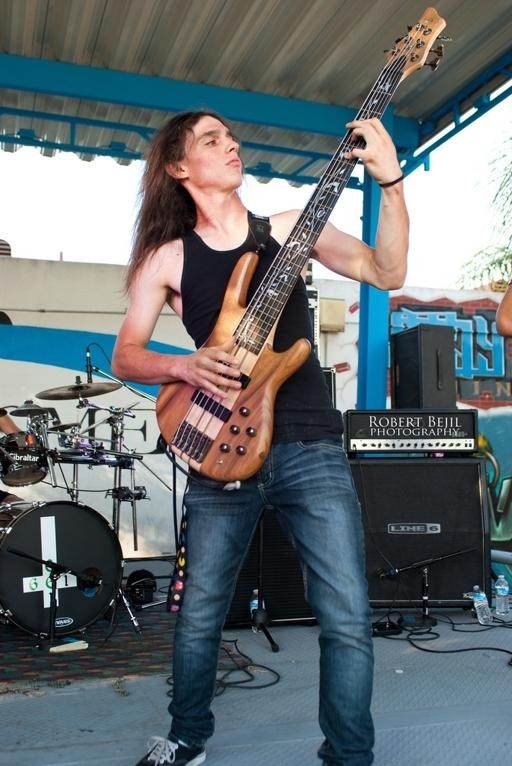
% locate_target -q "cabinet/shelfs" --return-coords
[471,584,494,625]
[494,573,510,617]
[248,588,268,635]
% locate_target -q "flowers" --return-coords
[85,349,95,384]
[376,567,390,580]
[85,575,98,587]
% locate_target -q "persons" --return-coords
[0,408,22,436]
[111,111,410,766]
[495,279,512,337]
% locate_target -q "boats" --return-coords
[222,509,320,625]
[347,457,492,609]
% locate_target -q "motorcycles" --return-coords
[222,509,320,649]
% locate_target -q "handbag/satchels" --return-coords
[379,174,403,188]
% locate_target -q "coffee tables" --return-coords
[47,423,80,433]
[9,403,46,417]
[35,382,122,399]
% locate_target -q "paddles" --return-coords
[0,582,265,698]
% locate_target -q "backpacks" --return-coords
[0,431,47,487]
[0,500,126,641]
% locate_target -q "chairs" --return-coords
[135,733,206,766]
